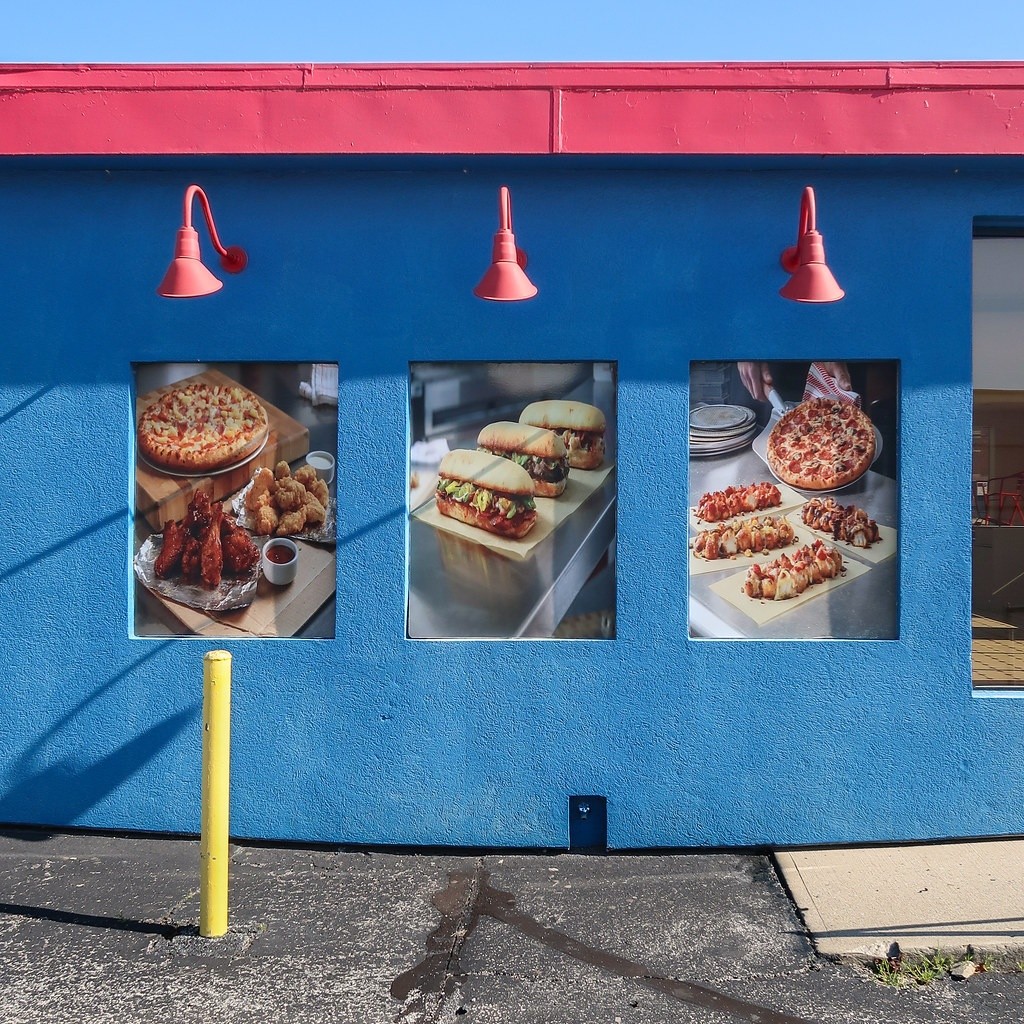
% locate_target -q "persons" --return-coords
[728,360,889,477]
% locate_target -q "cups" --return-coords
[306,451,335,484]
[262,537,298,585]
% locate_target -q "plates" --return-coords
[767,439,883,493]
[689,401,756,456]
[136,407,269,476]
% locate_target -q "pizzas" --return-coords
[767,396,875,489]
[141,380,268,471]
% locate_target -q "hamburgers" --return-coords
[433,399,605,539]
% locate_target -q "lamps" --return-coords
[779,187,846,303]
[155,183,247,299]
[473,185,540,300]
